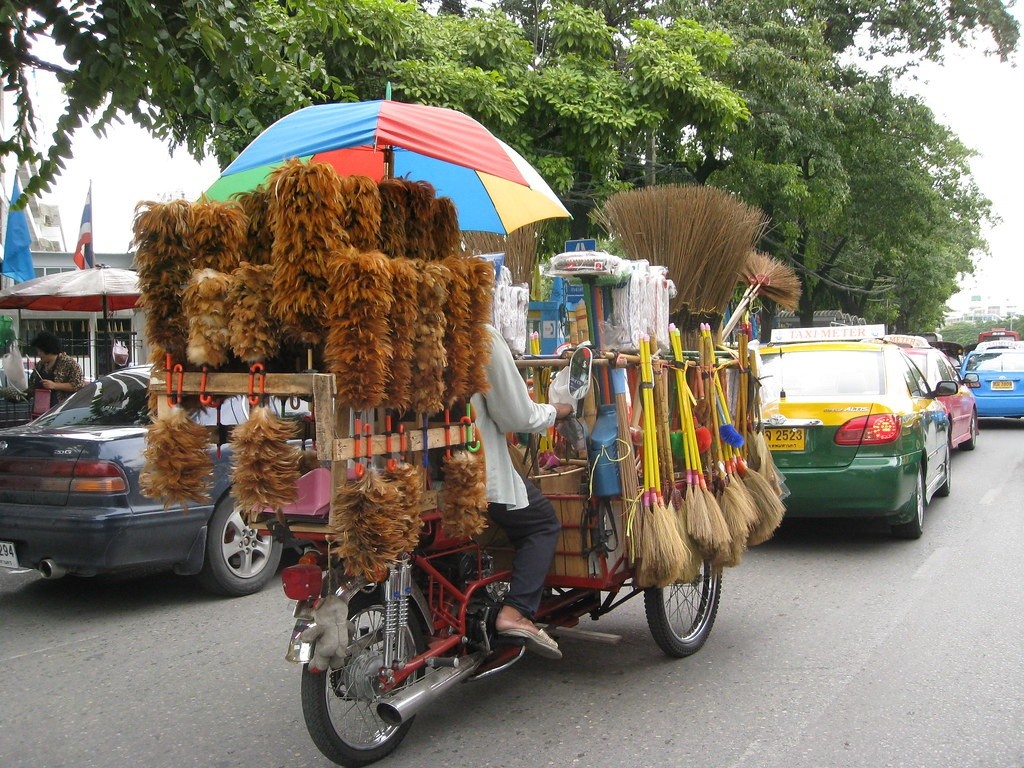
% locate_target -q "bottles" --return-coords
[590,404,622,497]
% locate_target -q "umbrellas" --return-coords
[198,80,573,236]
[0,263,145,374]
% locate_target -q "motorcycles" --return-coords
[280,409,722,768]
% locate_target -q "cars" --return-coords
[976,341,1024,350]
[882,333,980,452]
[742,321,958,539]
[0,364,318,599]
[961,349,1024,418]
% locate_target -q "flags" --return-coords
[73,189,93,269]
[2,175,36,283]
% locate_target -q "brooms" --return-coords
[544,181,804,590]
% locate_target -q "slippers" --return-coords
[497,627,563,660]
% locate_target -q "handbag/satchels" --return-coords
[112,339,129,367]
[2,340,28,392]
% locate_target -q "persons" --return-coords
[469,323,574,653]
[16,331,84,420]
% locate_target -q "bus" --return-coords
[978,327,1019,343]
